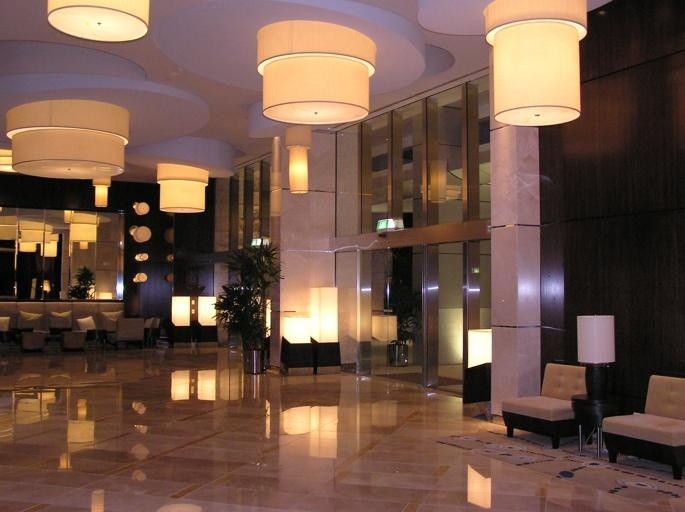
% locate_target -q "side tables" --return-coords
[571,395,623,459]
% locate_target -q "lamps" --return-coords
[576,314,617,398]
[256,20,377,125]
[0,369,400,512]
[482,1,588,127]
[284,125,312,194]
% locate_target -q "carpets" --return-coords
[436,426,684,512]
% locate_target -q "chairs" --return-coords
[501,362,589,449]
[602,374,684,480]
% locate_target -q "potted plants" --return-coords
[211,237,289,375]
